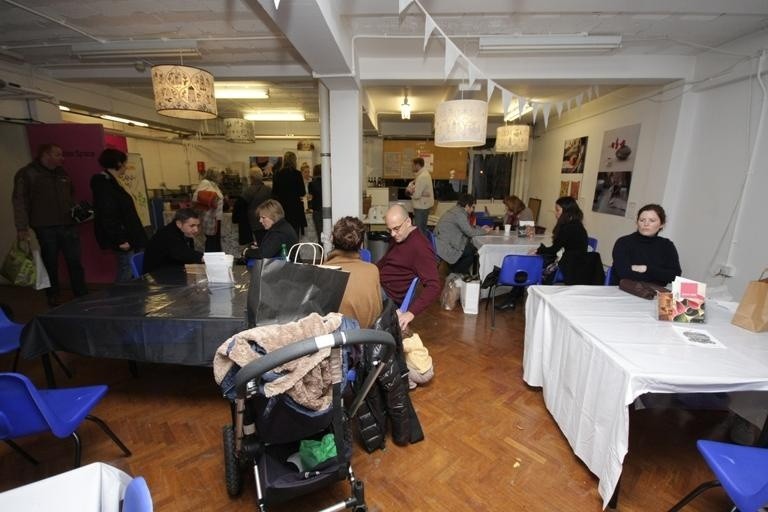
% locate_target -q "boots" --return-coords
[494,295,516,312]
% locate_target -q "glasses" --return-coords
[386,217,408,234]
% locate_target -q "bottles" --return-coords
[280,243,289,259]
[368,176,385,187]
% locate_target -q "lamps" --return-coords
[400,86,410,119]
[243,112,306,121]
[478,35,622,53]
[224,102,256,144]
[434,80,489,148]
[72,41,201,59]
[151,39,218,120]
[504,101,533,122]
[496,125,530,152]
[215,88,270,99]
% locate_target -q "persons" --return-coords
[410,157,435,232]
[231,151,321,260]
[11,141,89,307]
[399,325,435,389]
[320,215,383,330]
[144,208,204,276]
[375,204,443,331]
[433,193,493,274]
[405,180,431,198]
[503,196,534,231]
[191,166,225,252]
[494,196,586,311]
[608,204,682,288]
[90,148,149,283]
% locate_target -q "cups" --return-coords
[504,225,512,236]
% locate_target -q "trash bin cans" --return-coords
[367,230,391,264]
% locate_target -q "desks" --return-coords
[524,285,768,511]
[20,264,252,387]
[471,231,593,299]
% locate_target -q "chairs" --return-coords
[0,309,72,377]
[485,254,543,331]
[0,372,132,469]
[552,237,614,285]
[667,440,768,512]
[399,276,421,313]
[130,252,144,278]
[425,229,443,269]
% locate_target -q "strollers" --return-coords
[204,311,397,510]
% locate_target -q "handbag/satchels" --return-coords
[730,266,767,334]
[246,242,351,330]
[1,230,52,291]
[460,274,482,315]
